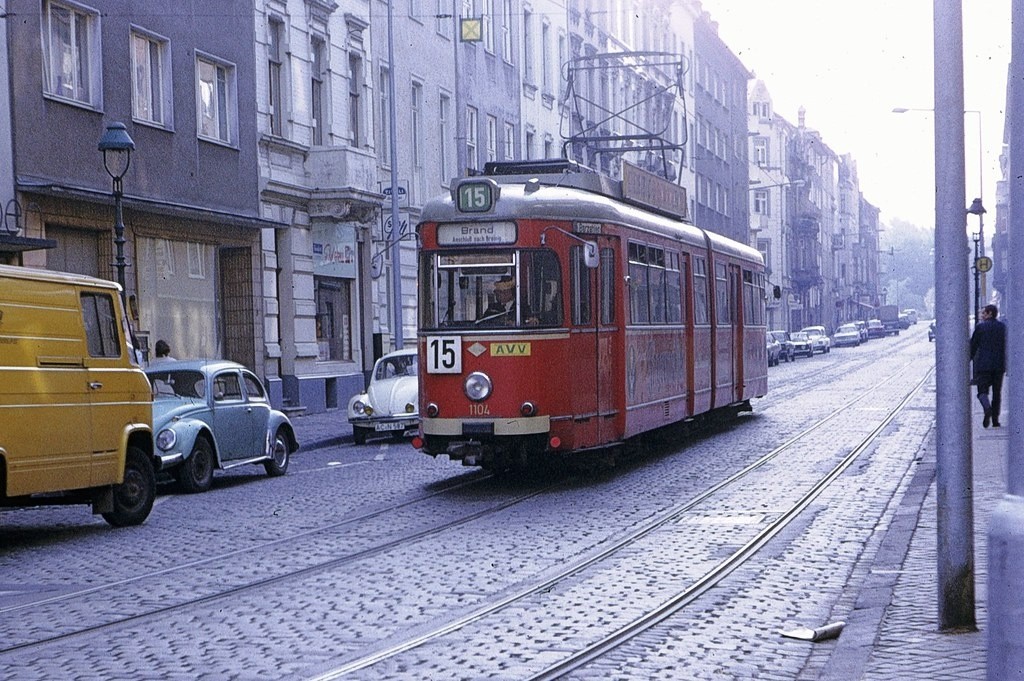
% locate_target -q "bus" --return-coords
[410,156,782,468]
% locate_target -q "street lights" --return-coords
[744,178,806,248]
[965,198,987,385]
[97,120,137,315]
[892,106,988,305]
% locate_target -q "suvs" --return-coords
[800,325,831,354]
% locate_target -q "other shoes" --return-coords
[992,422,1000,427]
[983,407,993,427]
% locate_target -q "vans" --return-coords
[0,261,163,528]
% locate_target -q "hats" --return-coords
[494,275,515,289]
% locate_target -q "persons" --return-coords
[148,340,178,396]
[483,277,540,326]
[970,305,1007,427]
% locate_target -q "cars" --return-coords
[770,330,796,362]
[928,320,936,342]
[789,331,814,358]
[144,359,300,495]
[347,347,418,444]
[765,332,782,367]
[833,304,921,348]
